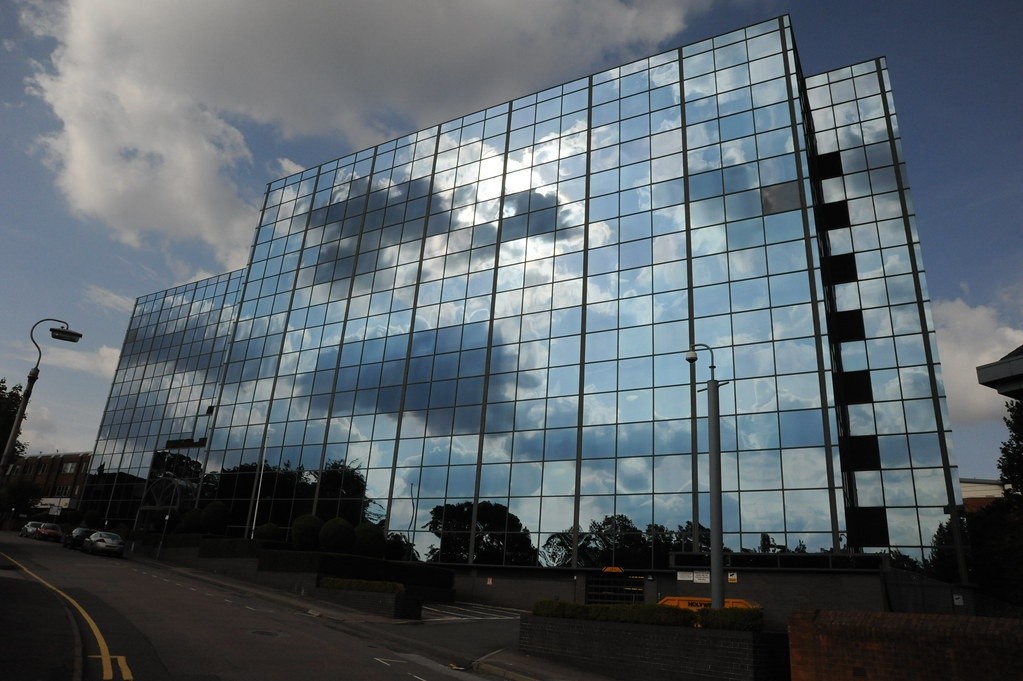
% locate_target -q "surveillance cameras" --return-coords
[685,352,698,364]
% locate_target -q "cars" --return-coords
[18,521,45,539]
[63,527,100,551]
[82,532,127,559]
[33,523,62,542]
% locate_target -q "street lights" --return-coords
[0,318,84,474]
[687,343,731,616]
